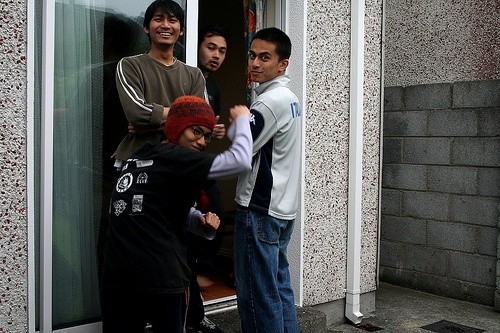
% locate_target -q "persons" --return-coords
[198,28,229,141]
[224,27,302,332]
[111,0,212,171]
[99,94,254,333]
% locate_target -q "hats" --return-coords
[164,95,216,143]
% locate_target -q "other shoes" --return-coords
[199,316,221,333]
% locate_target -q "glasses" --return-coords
[190,126,212,143]
